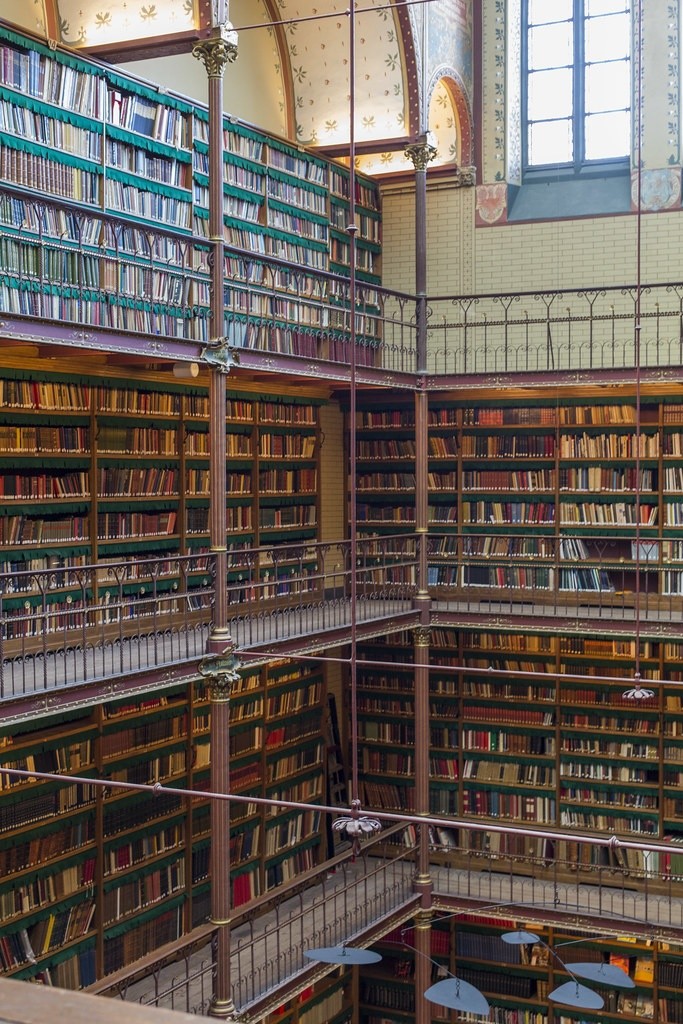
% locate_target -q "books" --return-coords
[0,660,323,993]
[358,912,683,1024]
[270,983,353,1024]
[223,128,381,367]
[345,626,683,883]
[349,402,683,596]
[0,39,210,343]
[0,379,324,640]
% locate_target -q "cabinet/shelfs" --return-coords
[0,358,334,661]
[344,621,683,895]
[343,390,683,615]
[235,909,683,1024]
[0,650,348,998]
[0,16,383,372]
